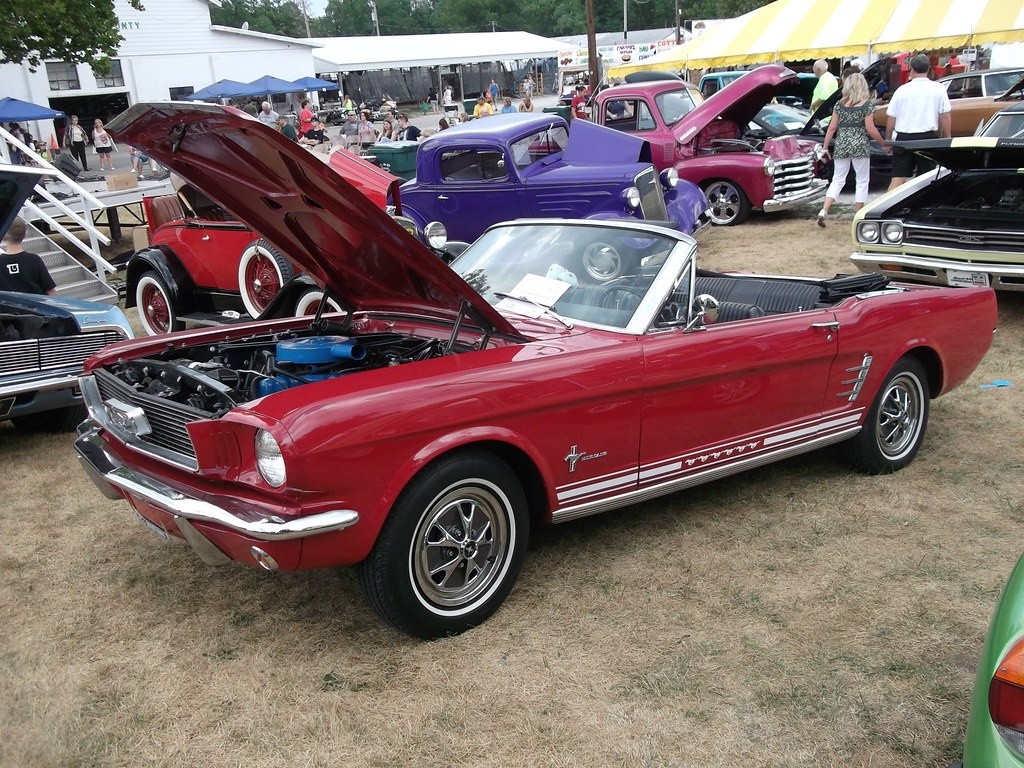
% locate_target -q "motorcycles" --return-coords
[326,99,362,126]
[358,93,409,124]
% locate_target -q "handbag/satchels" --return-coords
[108,134,118,152]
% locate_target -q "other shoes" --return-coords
[109,168,115,170]
[84,170,90,171]
[816,209,827,228]
[495,109,498,111]
[139,175,144,178]
[100,168,104,172]
[152,168,158,172]
[131,169,136,173]
[142,171,145,172]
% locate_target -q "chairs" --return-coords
[152,194,180,226]
[962,87,981,98]
[571,287,641,311]
[679,301,765,325]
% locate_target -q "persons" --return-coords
[342,95,353,110]
[817,73,888,227]
[0,123,53,179]
[599,82,633,121]
[0,218,57,297]
[885,54,951,192]
[487,78,500,111]
[62,115,90,172]
[523,75,533,99]
[809,59,859,128]
[421,85,453,115]
[339,110,430,147]
[128,145,160,179]
[571,85,589,120]
[243,93,323,147]
[92,119,118,171]
[438,97,534,131]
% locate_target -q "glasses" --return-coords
[347,115,354,116]
[39,144,46,146]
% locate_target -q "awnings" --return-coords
[608,0,1024,78]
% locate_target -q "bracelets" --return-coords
[821,150,829,152]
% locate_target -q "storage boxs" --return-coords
[105,172,138,191]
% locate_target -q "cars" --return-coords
[73,98,997,639]
[526,64,829,228]
[540,51,1024,191]
[390,112,713,286]
[0,162,136,436]
[123,146,471,336]
[851,99,1024,293]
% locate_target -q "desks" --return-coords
[17,179,176,241]
[316,108,346,123]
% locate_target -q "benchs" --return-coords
[605,120,650,132]
[671,277,821,316]
[446,161,486,182]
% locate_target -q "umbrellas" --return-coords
[186,76,340,102]
[0,97,65,122]
